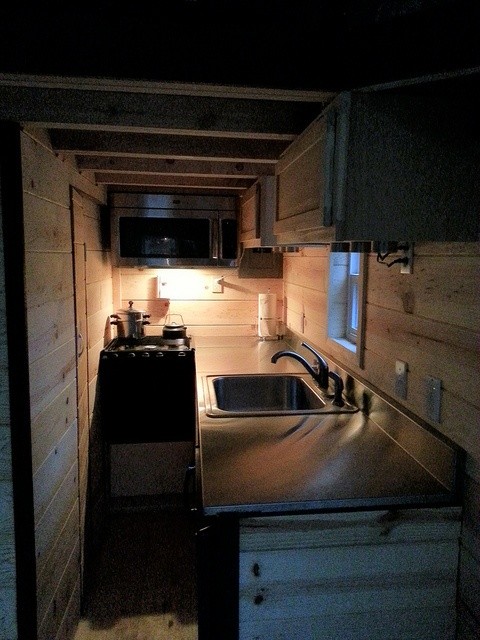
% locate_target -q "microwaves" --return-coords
[109,206,218,269]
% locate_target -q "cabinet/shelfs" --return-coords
[275,107,414,245]
[238,175,273,248]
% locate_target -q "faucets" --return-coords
[271,341,330,390]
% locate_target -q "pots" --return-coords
[109,300,151,338]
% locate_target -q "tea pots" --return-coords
[162,313,187,339]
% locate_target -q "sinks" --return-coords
[199,372,359,417]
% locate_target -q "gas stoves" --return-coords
[98,336,196,363]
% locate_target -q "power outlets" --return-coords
[399,255,412,275]
